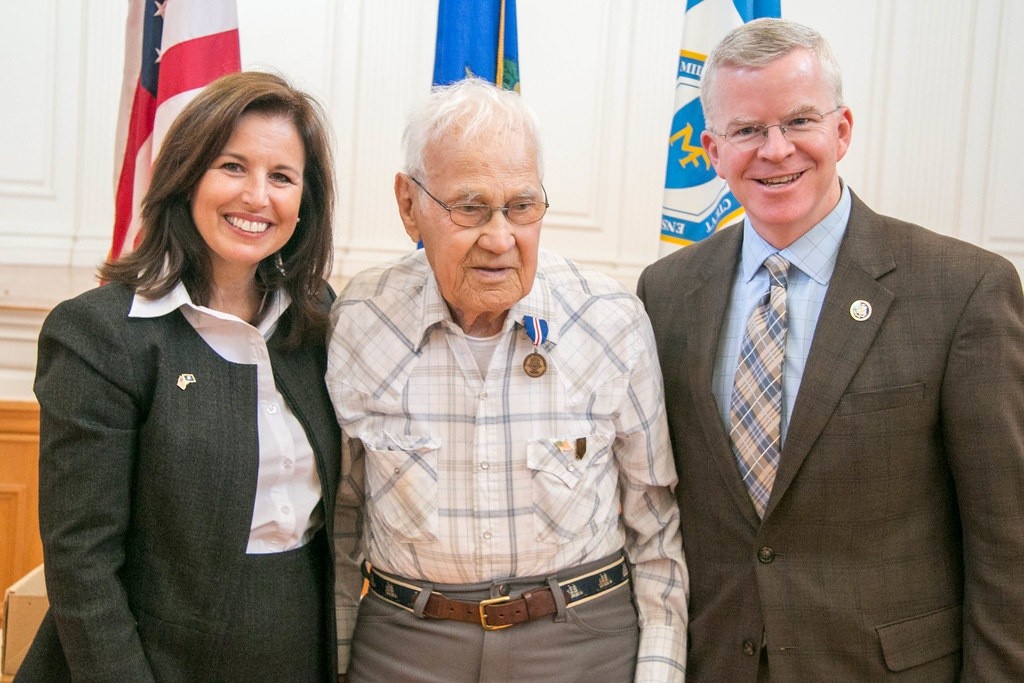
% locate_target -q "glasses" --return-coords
[411,176,550,228]
[710,107,841,152]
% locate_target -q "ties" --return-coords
[728,253,791,521]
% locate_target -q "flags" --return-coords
[637,17,1024,682]
[96,0,242,279]
[432,0,523,102]
[660,0,784,261]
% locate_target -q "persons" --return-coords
[327,79,688,683]
[12,73,343,683]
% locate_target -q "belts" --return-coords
[369,557,631,631]
